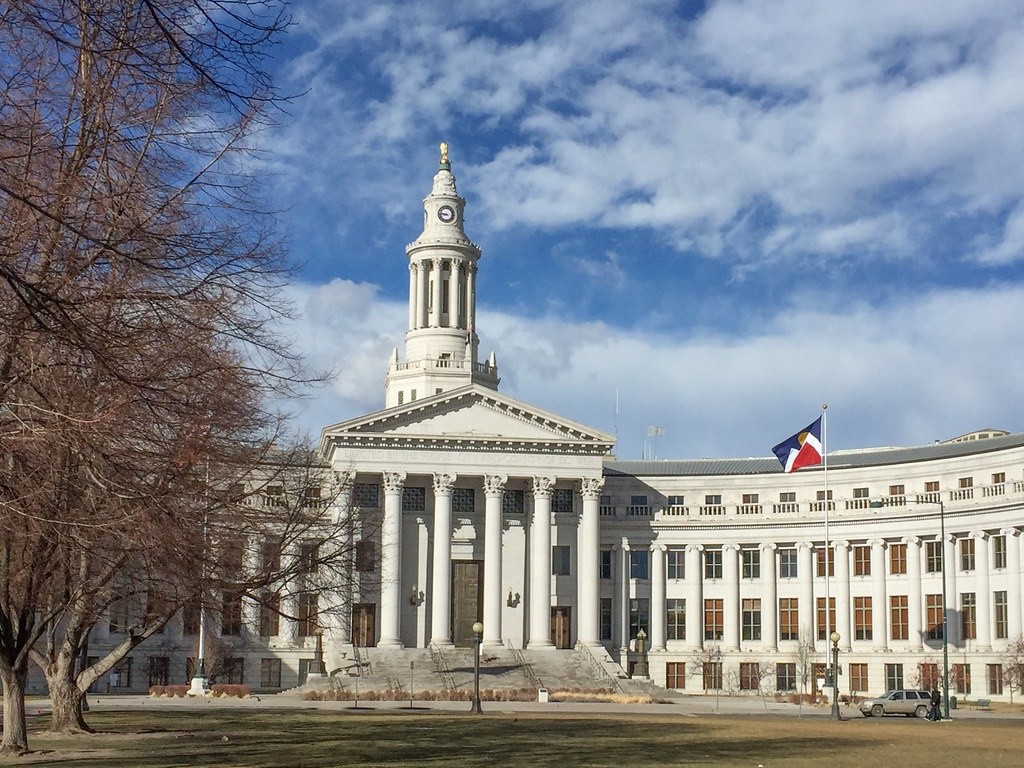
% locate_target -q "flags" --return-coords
[771,414,824,474]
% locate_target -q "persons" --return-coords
[924,688,942,722]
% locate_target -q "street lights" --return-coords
[868,500,952,724]
[828,632,843,722]
[470,621,484,714]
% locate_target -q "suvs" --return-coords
[858,690,933,718]
